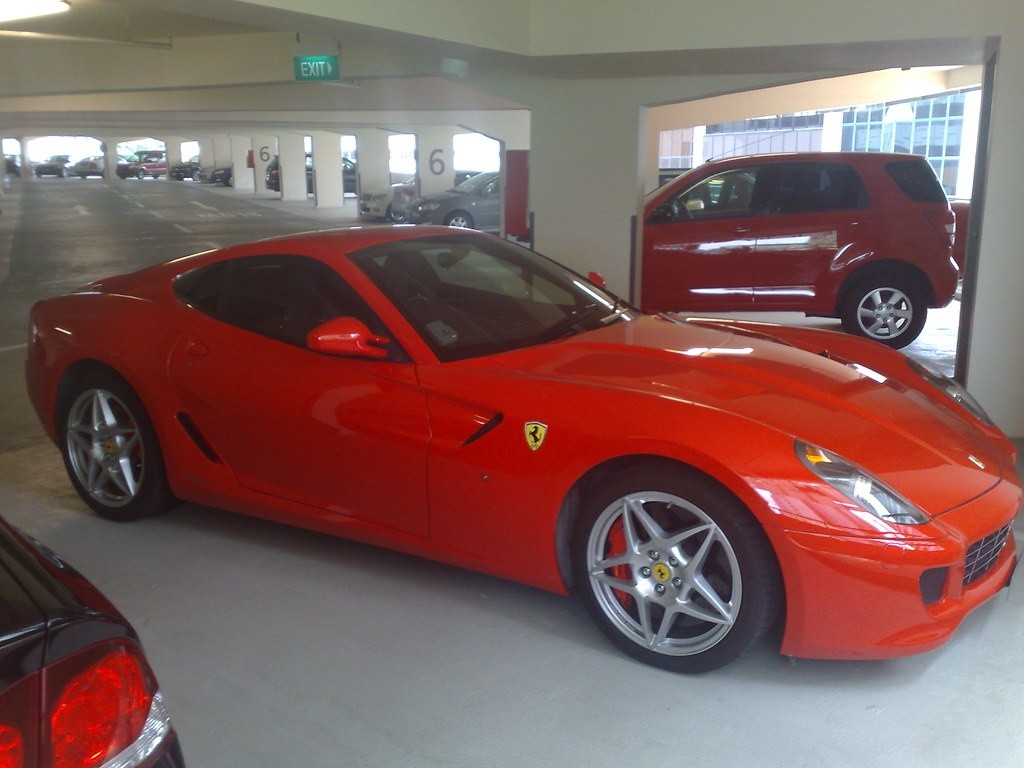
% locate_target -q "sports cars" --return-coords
[24,225,1024,674]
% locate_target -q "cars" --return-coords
[199,166,216,180]
[74,156,126,179]
[0,516,187,767]
[3,154,34,176]
[358,190,405,225]
[265,153,356,193]
[413,172,503,231]
[115,151,167,179]
[170,155,200,181]
[641,152,959,350]
[34,154,80,178]
[658,167,756,203]
[391,170,482,216]
[212,165,233,187]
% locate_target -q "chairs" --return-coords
[750,170,780,215]
